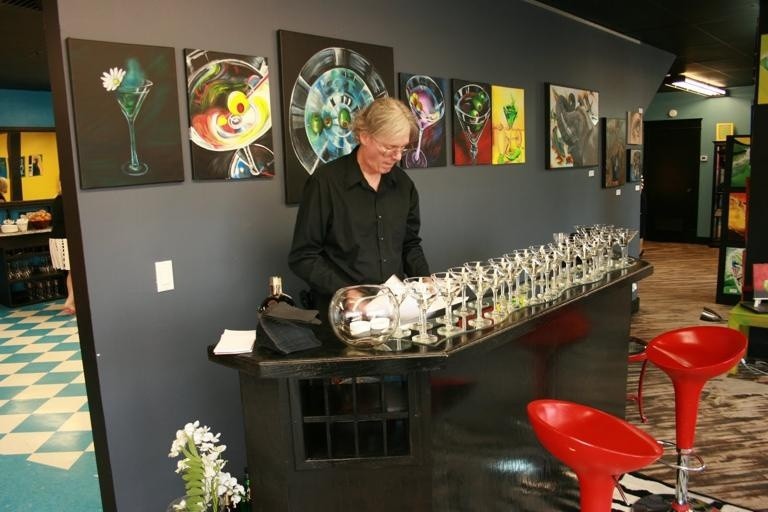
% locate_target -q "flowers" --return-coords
[159,415,252,512]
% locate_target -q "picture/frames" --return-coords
[714,121,734,141]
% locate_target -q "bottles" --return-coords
[8,255,57,280]
[24,280,58,300]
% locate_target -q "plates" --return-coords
[2,225,18,232]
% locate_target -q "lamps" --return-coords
[664,72,733,102]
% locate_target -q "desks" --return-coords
[722,300,767,378]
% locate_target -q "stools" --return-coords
[644,324,751,512]
[523,397,665,512]
[620,335,653,429]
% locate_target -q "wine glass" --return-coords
[455,84,492,160]
[502,104,523,161]
[103,81,153,177]
[186,51,275,179]
[404,75,445,168]
[389,221,640,346]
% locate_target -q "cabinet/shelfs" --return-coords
[0,226,70,311]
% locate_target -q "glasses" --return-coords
[381,148,410,157]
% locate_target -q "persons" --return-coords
[0,177,8,203]
[288,96,431,333]
[33,158,40,176]
[48,191,77,316]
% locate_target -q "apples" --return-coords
[26,210,52,222]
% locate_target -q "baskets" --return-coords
[29,220,52,229]
